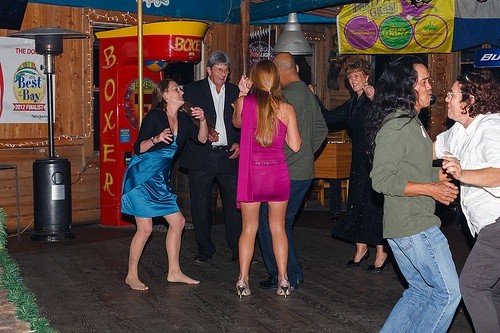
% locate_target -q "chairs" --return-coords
[303,129,353,209]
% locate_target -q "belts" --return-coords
[211,145,229,151]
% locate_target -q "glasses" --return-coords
[447,92,467,99]
[211,68,230,74]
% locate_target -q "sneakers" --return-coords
[235,257,259,264]
[290,279,303,292]
[194,255,213,263]
[258,280,278,289]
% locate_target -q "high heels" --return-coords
[277,280,291,298]
[346,249,370,267]
[236,280,251,299]
[366,254,390,272]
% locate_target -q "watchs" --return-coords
[151,136,156,145]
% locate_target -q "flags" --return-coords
[336,0,454,54]
[452,0,500,52]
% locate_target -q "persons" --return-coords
[121,77,208,290]
[433,68,500,333]
[232,59,302,299]
[308,58,390,272]
[363,55,462,333]
[182,50,258,264]
[258,52,329,289]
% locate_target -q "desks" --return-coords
[314,144,353,221]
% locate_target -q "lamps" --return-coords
[273,12,313,55]
